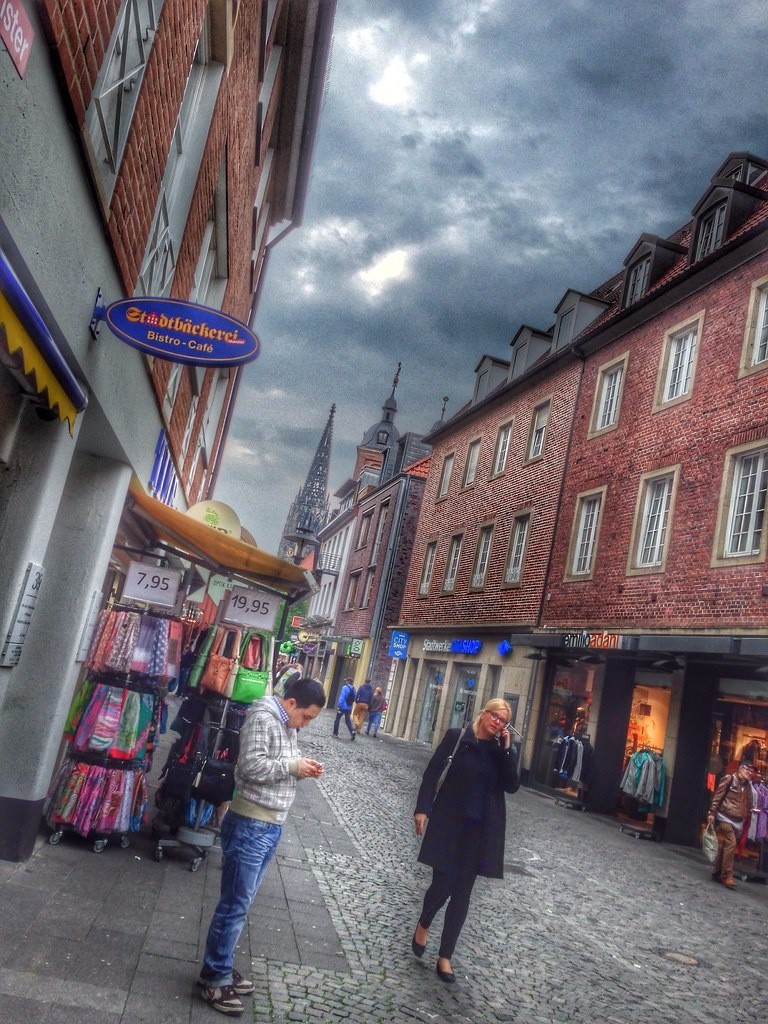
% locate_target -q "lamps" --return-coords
[523,653,547,661]
[652,660,685,673]
[580,655,604,665]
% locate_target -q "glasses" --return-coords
[486,710,508,727]
[742,767,754,775]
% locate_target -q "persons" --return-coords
[412,698,520,982]
[708,762,758,888]
[365,687,388,737]
[333,677,357,740]
[353,678,374,734]
[196,678,326,1015]
[275,660,303,733]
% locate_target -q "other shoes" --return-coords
[720,876,737,887]
[351,731,356,741]
[374,734,376,737]
[365,731,369,735]
[354,725,359,730]
[412,920,427,958]
[331,733,338,738]
[436,958,456,983]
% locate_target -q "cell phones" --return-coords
[504,722,511,730]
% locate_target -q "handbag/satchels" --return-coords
[187,625,273,705]
[155,716,242,824]
[430,727,466,802]
[703,821,718,864]
[383,698,387,710]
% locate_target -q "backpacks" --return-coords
[345,685,355,706]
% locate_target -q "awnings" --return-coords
[1,248,89,439]
[130,485,319,606]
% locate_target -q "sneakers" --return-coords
[233,969,256,995]
[196,979,245,1016]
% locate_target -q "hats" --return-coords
[740,760,758,772]
[344,677,353,682]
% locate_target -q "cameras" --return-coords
[314,763,324,767]
